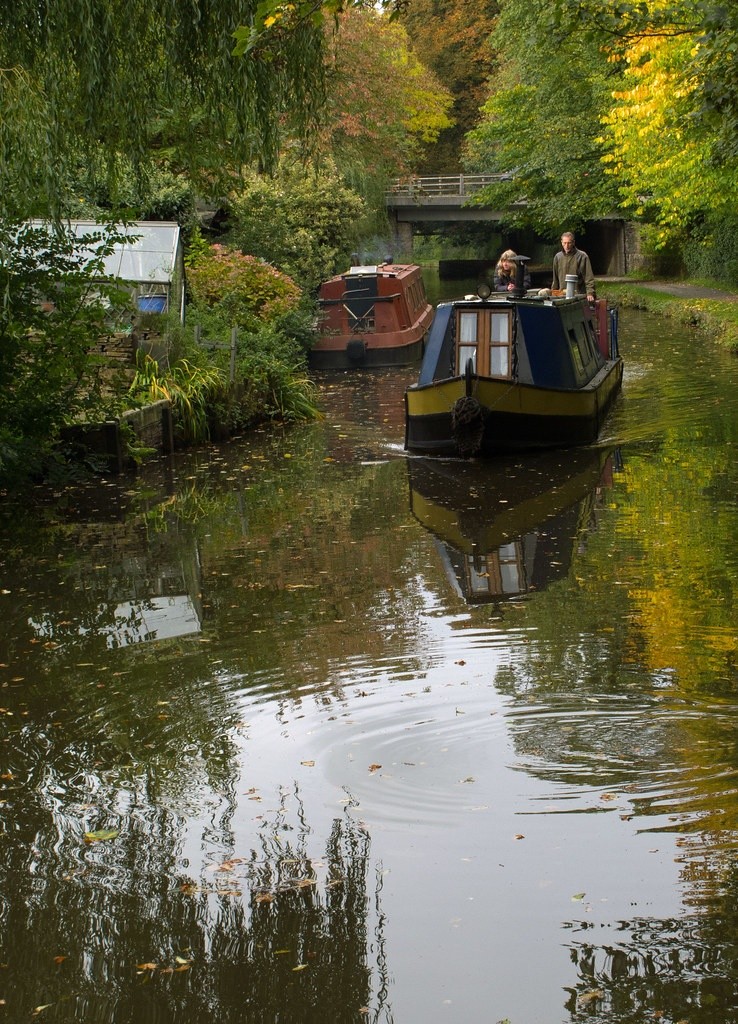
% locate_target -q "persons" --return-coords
[551,232,596,302]
[493,250,532,292]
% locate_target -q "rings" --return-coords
[510,289,511,290]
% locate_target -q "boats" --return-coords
[399,255,625,453]
[404,453,617,623]
[307,256,435,367]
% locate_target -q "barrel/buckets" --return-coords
[137,294,167,313]
